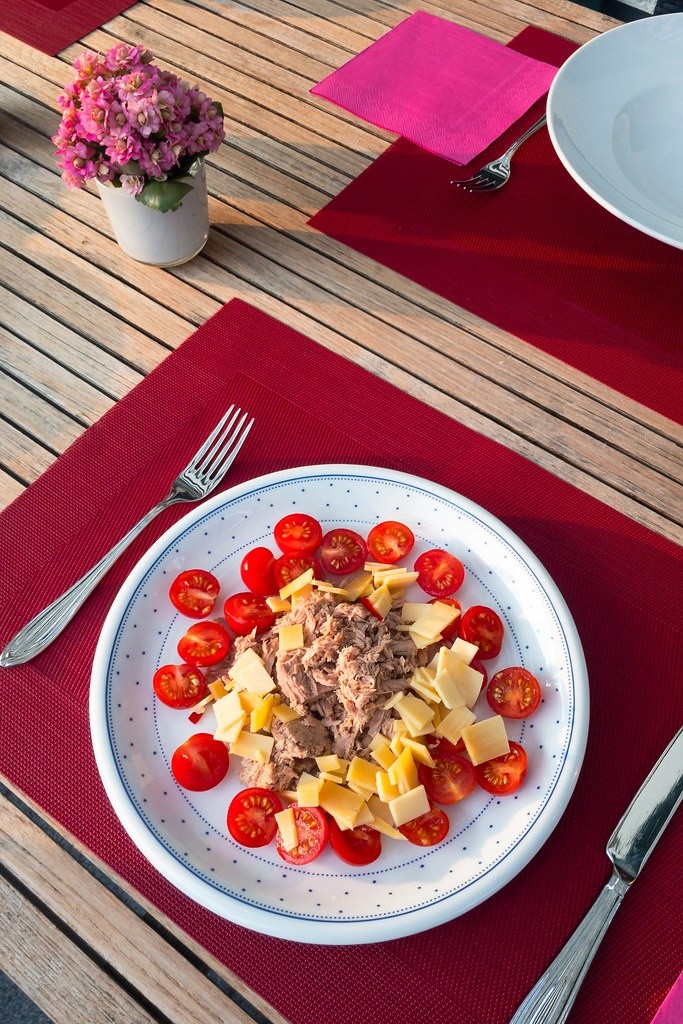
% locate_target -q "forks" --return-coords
[448,114,549,193]
[1,405,255,671]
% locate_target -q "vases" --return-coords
[95,153,211,267]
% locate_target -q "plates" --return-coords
[87,463,589,947]
[545,12,683,250]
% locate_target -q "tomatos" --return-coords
[153,514,543,866]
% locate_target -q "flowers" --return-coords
[52,44,226,213]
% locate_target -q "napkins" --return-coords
[308,9,559,167]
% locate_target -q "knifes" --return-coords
[505,726,683,1024]
